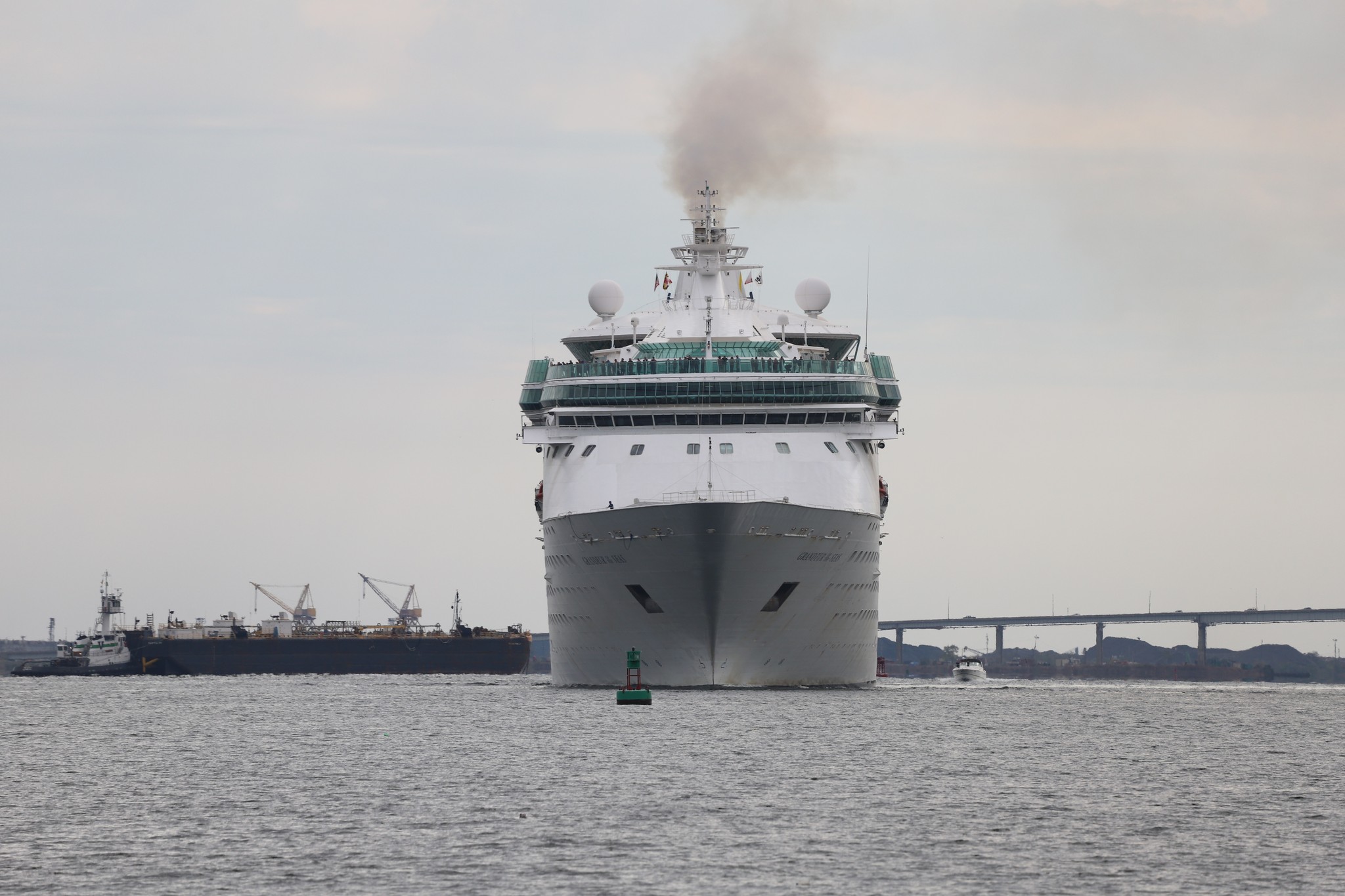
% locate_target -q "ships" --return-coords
[515,180,902,688]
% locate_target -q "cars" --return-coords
[1245,608,1257,612]
[1072,613,1080,616]
[1304,607,1311,609]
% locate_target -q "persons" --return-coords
[820,357,828,373]
[551,356,657,377]
[751,356,785,373]
[717,355,741,373]
[844,357,856,374]
[864,360,868,362]
[665,355,706,374]
[830,358,840,374]
[807,356,814,373]
[784,356,804,373]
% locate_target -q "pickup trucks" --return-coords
[963,616,976,618]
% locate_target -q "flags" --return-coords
[654,273,660,292]
[663,272,672,290]
[754,270,763,286]
[744,270,753,285]
[739,271,743,293]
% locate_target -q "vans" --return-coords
[1173,610,1182,613]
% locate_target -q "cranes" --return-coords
[249,581,316,632]
[357,572,422,637]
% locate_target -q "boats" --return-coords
[120,625,532,676]
[56,569,130,671]
[952,657,986,682]
[10,656,90,676]
